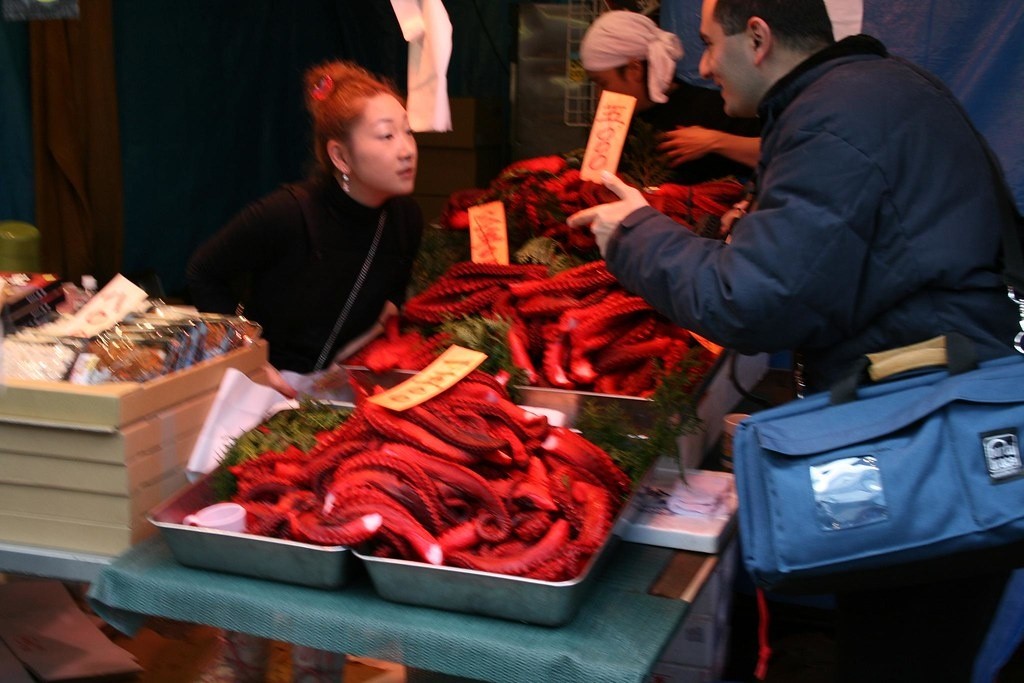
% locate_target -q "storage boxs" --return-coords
[147,399,351,587]
[345,429,652,626]
[511,367,656,424]
[404,92,506,231]
[331,311,422,387]
[0,336,269,559]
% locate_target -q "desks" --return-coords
[88,476,739,683]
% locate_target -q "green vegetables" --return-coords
[208,398,351,498]
[519,237,586,272]
[575,352,710,480]
[430,315,533,405]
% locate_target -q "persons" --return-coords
[581,11,762,187]
[568,0,1024,683]
[188,59,418,374]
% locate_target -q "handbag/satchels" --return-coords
[733,334,1023,582]
